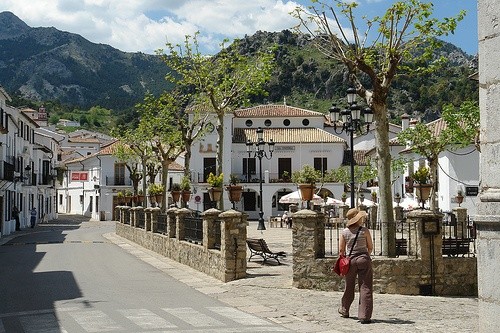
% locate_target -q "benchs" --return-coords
[396,239,412,255]
[246,238,286,266]
[442,239,471,258]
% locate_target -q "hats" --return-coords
[344,208,367,227]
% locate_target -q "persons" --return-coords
[337,208,373,323]
[30,208,37,228]
[283,211,292,228]
[12,206,21,231]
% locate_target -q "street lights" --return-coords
[328,87,374,208]
[245,126,276,228]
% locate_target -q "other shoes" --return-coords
[360,318,371,323]
[338,307,350,318]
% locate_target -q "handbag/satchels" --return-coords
[334,255,350,276]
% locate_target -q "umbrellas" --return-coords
[279,190,321,203]
[345,197,378,207]
[321,197,342,207]
[399,198,429,209]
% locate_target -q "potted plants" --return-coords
[112,166,433,202]
